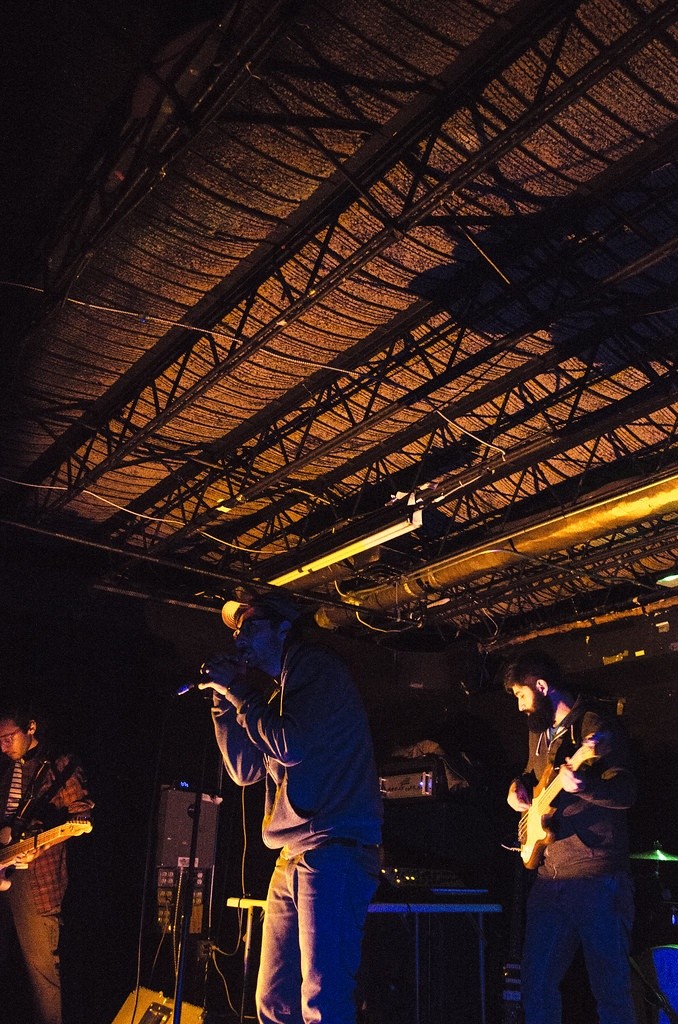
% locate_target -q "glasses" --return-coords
[0,727,22,747]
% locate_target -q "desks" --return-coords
[225,896,504,1024]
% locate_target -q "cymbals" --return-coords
[629,839,678,864]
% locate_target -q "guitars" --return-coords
[518,733,601,869]
[0,817,92,891]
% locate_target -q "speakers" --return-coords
[111,985,215,1024]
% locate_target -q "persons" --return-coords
[0,707,96,1024]
[503,650,635,1024]
[198,593,383,1024]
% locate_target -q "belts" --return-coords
[322,839,380,848]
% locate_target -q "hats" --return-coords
[222,592,302,630]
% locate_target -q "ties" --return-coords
[5,759,22,818]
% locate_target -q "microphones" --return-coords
[176,678,201,696]
